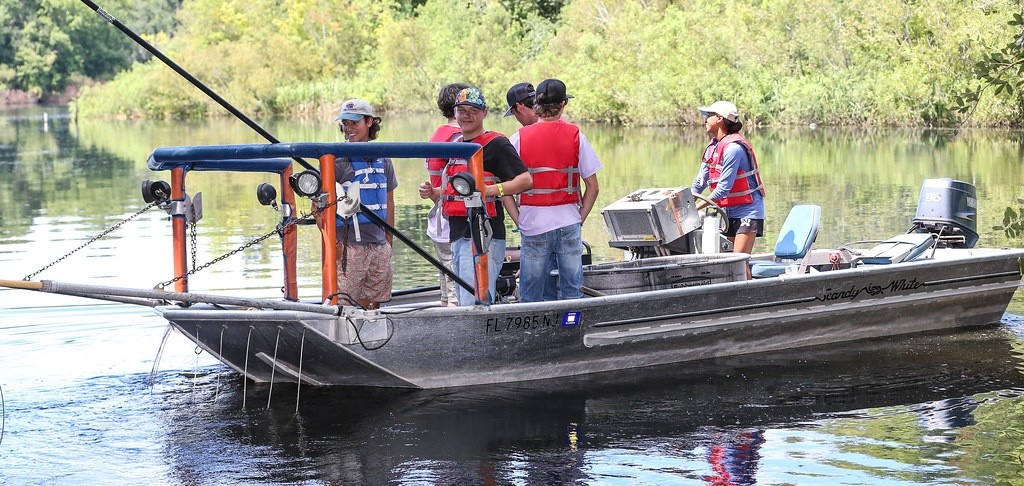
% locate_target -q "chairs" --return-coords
[748,204,821,278]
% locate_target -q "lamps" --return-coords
[450,169,483,208]
[256,181,290,217]
[140,179,175,215]
[297,169,328,210]
[288,173,306,198]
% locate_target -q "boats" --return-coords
[0,143,1024,411]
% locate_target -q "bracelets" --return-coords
[497,183,504,198]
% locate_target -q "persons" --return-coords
[691,100,766,281]
[504,83,559,301]
[419,88,533,306]
[424,83,471,307]
[502,78,602,303]
[310,98,398,309]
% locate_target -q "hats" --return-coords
[452,87,485,110]
[535,79,575,105]
[698,101,738,124]
[504,82,535,118]
[335,98,372,122]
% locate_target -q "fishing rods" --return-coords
[78,1,474,295]
